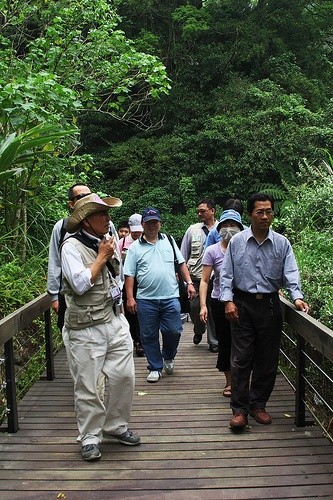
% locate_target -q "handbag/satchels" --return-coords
[178,280,191,314]
[56,289,69,333]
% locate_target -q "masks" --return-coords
[218,226,241,243]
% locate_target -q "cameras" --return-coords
[115,298,123,315]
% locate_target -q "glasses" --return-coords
[73,193,91,201]
[254,210,274,217]
[197,208,210,214]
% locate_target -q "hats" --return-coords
[128,213,144,232]
[65,193,123,234]
[142,207,162,223]
[216,208,244,230]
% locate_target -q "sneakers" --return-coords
[145,369,162,383]
[163,357,175,375]
[101,427,141,447]
[80,443,102,461]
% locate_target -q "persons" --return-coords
[47,183,283,397]
[220,193,309,427]
[59,193,141,461]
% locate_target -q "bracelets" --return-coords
[187,281,194,285]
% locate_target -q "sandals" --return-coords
[222,383,232,397]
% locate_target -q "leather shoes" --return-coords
[229,411,248,428]
[248,407,272,425]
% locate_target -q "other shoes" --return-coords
[192,333,203,344]
[208,343,220,353]
[134,336,145,355]
